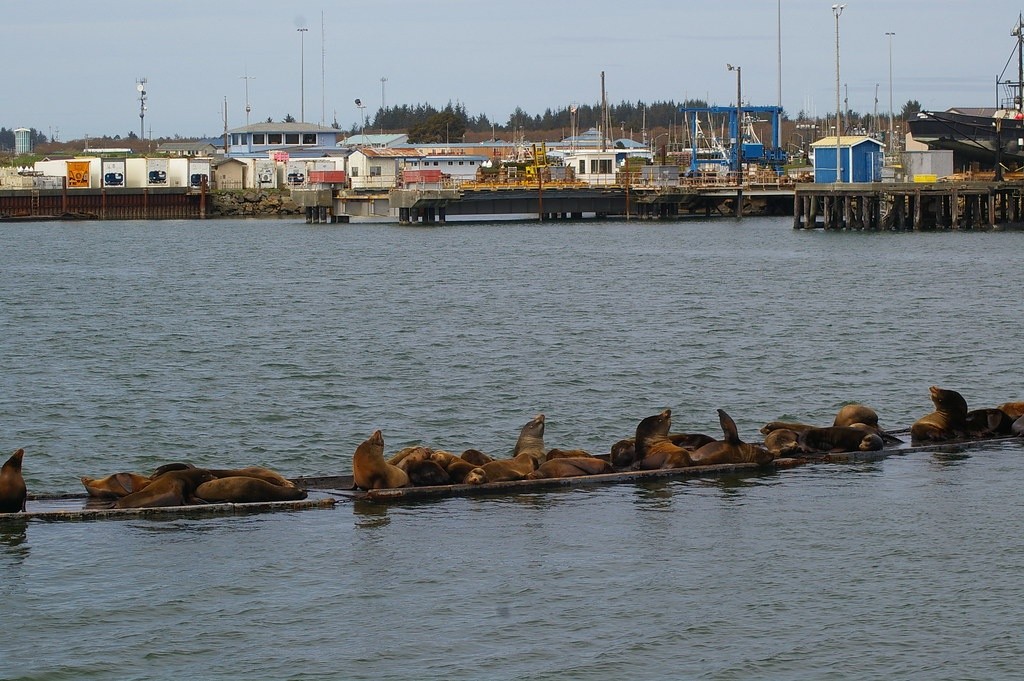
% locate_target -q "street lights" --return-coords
[297,28,309,123]
[831,4,846,181]
[379,77,390,112]
[240,76,256,123]
[884,32,901,156]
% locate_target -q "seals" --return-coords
[81,463,308,509]
[352,402,902,492]
[1,449,27,513]
[911,386,1024,440]
